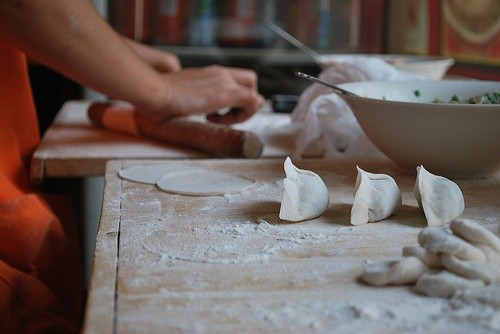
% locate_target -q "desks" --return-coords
[85,155,500,334]
[29,100,387,179]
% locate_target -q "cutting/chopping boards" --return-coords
[32,100,500,333]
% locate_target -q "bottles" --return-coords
[100,1,351,55]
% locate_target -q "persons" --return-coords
[1,1,267,334]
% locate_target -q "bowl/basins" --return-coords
[315,52,455,82]
[332,78,500,190]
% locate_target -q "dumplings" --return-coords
[413,164,465,227]
[349,163,402,225]
[279,155,329,221]
